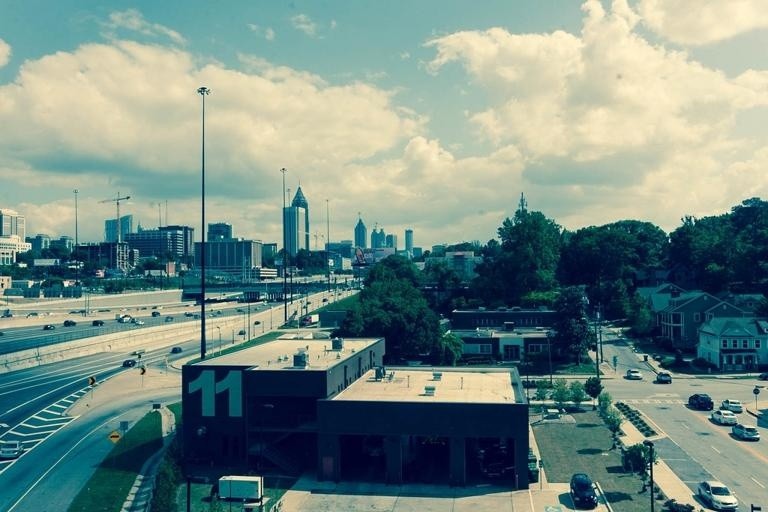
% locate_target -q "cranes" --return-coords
[96,191,130,243]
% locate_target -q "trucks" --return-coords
[210,475,263,500]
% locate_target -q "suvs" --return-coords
[569,473,599,509]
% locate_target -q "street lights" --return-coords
[72,188,80,286]
[195,86,210,360]
[593,304,604,379]
[641,439,655,512]
[241,237,245,282]
[278,167,362,323]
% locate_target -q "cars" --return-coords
[165,311,200,322]
[43,324,55,330]
[711,409,739,424]
[627,368,643,380]
[64,304,161,326]
[722,398,743,412]
[122,346,145,367]
[254,321,260,325]
[0,309,37,336]
[689,394,713,411]
[238,330,246,335]
[171,347,182,353]
[1,440,24,460]
[656,371,672,384]
[732,423,761,441]
[697,480,740,511]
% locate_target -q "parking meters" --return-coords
[539,460,543,490]
